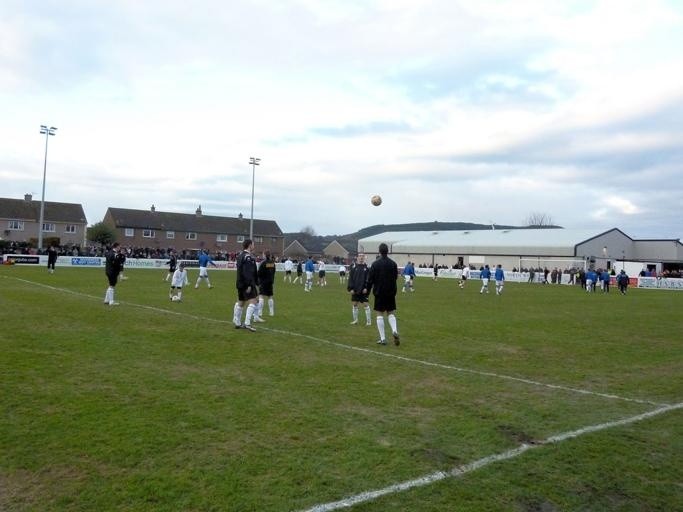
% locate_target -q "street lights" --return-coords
[39,123,58,249]
[247,156,262,243]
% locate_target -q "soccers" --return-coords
[171,296,178,301]
[372,195,382,206]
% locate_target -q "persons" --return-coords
[233,240,279,330]
[366,243,400,345]
[104,243,121,306]
[118,248,128,280]
[166,251,177,280]
[347,251,371,325]
[170,264,187,302]
[195,249,216,289]
[209,251,238,262]
[282,255,347,291]
[1,240,203,261]
[401,261,656,296]
[46,245,57,273]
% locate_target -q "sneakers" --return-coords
[109,303,120,306]
[233,317,266,332]
[350,319,400,346]
